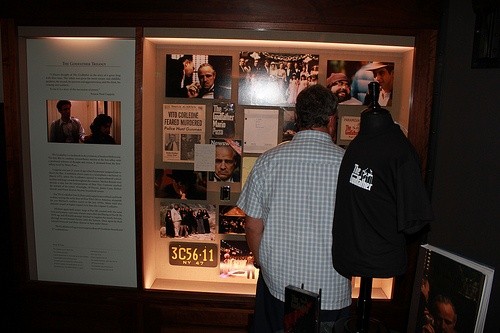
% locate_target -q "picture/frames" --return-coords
[472,4,500,69]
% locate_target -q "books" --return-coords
[408,243,494,333]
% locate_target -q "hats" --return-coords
[365,62,394,71]
[327,73,352,87]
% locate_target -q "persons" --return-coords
[187,138,193,158]
[164,204,210,237]
[236,84,352,333]
[365,60,394,107]
[157,170,206,200]
[327,73,363,105]
[246,251,255,280]
[210,143,238,182]
[224,249,230,275]
[83,113,117,145]
[188,63,229,99]
[219,219,246,233]
[418,276,456,333]
[238,57,318,104]
[165,134,178,151]
[49,100,84,143]
[166,55,200,98]
